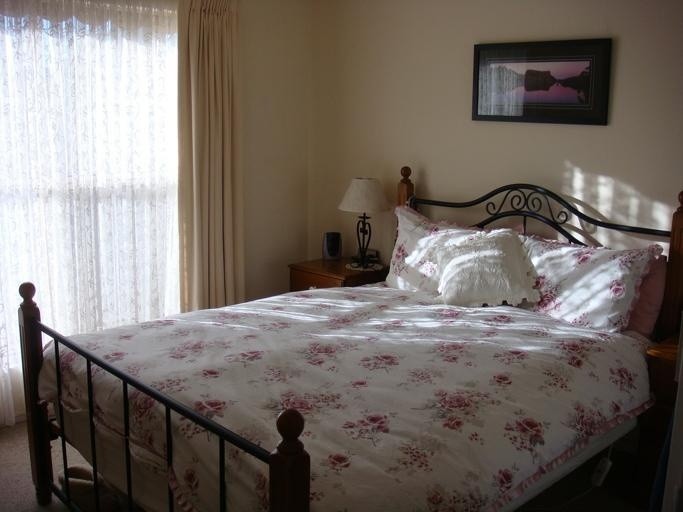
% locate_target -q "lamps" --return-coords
[336,177,391,272]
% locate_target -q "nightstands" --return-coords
[288,257,389,291]
[642,333,679,439]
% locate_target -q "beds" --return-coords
[17,164,683,511]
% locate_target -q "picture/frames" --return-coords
[472,38,615,126]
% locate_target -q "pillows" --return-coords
[384,203,664,335]
